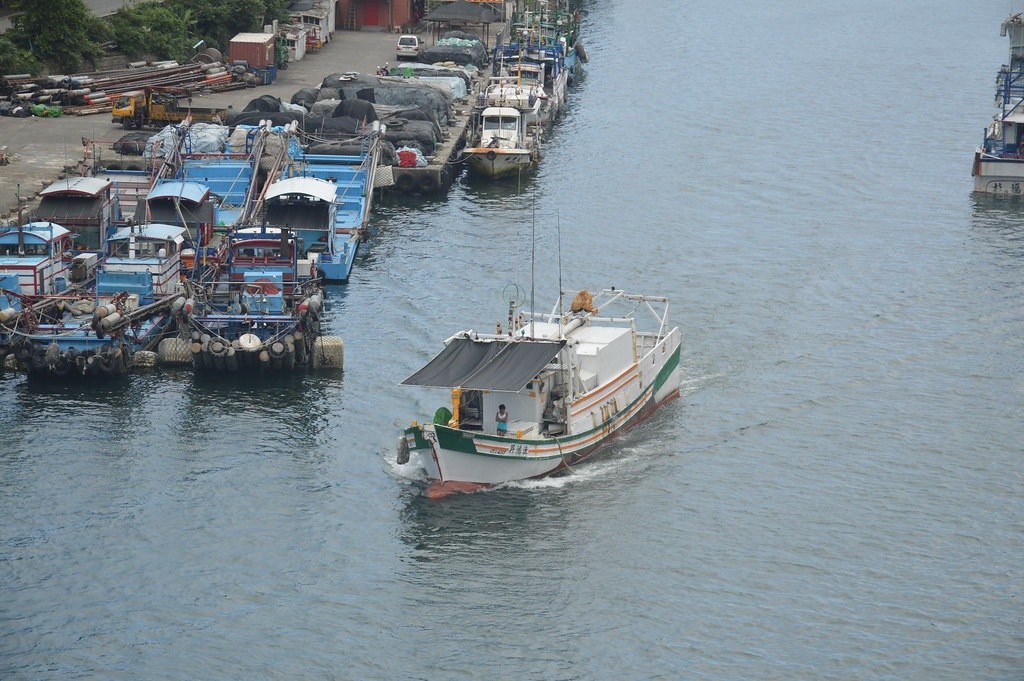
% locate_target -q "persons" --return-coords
[495,404,509,436]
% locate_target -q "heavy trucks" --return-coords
[111,86,227,130]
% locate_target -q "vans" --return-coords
[396,35,425,61]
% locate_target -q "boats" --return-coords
[396,179,684,499]
[14,112,272,380]
[456,0,589,181]
[971,2,1024,197]
[0,109,161,354]
[178,100,396,368]
